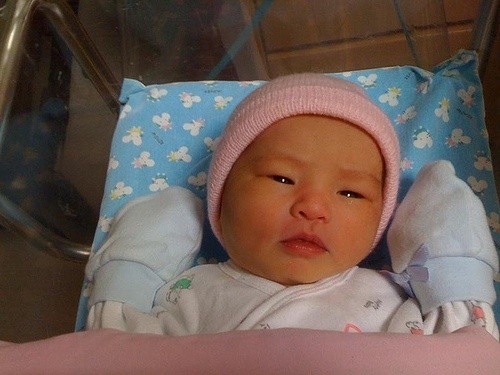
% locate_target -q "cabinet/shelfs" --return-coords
[207,0,500,85]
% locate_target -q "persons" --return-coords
[86,74,499,342]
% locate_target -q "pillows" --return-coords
[74,51,500,334]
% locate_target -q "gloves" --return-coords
[381,160,498,315]
[84,186,204,313]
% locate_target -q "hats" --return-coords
[208,73,400,255]
